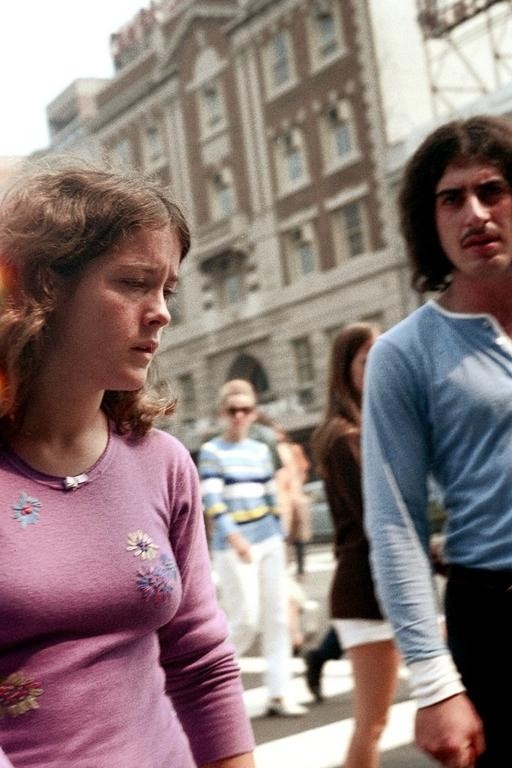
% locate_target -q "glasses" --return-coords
[222,406,255,417]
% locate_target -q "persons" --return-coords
[256,408,312,655]
[3,162,264,768]
[305,320,448,767]
[201,379,309,716]
[303,621,343,701]
[358,114,510,767]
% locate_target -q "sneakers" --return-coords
[263,636,325,718]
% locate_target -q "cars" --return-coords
[295,481,336,539]
